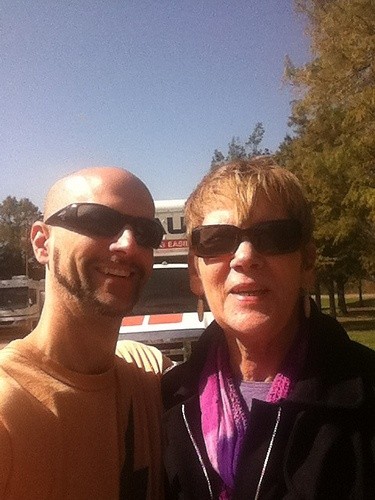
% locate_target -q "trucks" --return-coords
[118,200,214,364]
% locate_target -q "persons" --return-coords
[154,160,375,500]
[0,165,180,500]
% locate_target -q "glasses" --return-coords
[44,203,164,250]
[191,217,312,259]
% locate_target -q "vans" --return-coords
[0,275,41,332]
